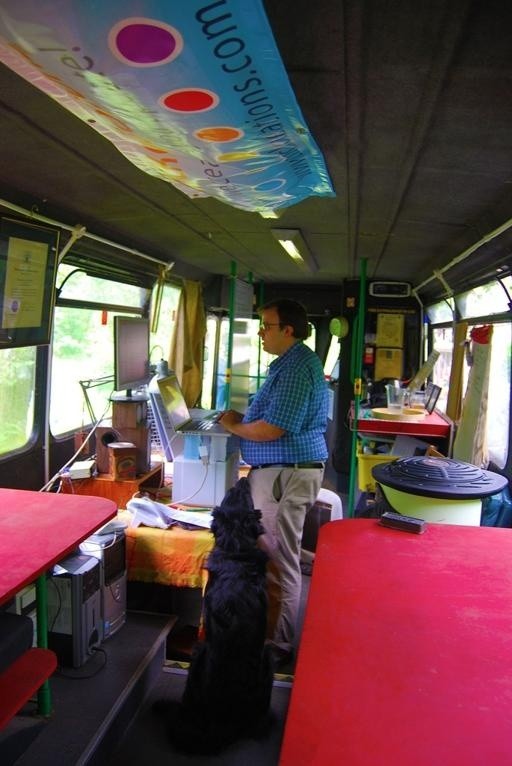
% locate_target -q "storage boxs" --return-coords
[354,439,419,492]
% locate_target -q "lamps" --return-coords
[77,358,174,424]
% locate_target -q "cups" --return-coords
[385,385,404,413]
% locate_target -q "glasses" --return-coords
[261,322,276,330]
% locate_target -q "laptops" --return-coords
[157,374,234,437]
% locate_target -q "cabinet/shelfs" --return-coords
[347,400,454,520]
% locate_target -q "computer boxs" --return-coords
[78,530,128,642]
[46,555,103,670]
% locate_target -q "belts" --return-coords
[252,463,323,468]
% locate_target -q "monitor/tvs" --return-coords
[299,488,343,576]
[108,316,151,401]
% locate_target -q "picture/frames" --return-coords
[426,385,441,413]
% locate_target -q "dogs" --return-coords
[151,476,278,761]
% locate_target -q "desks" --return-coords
[276,517,512,766]
[60,442,252,645]
[0,488,118,732]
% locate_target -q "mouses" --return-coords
[94,520,128,535]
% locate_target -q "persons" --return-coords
[211,302,328,670]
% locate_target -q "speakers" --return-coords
[96,420,152,474]
[111,401,147,430]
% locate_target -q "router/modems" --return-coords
[70,460,96,479]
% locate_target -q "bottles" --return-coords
[409,391,426,408]
